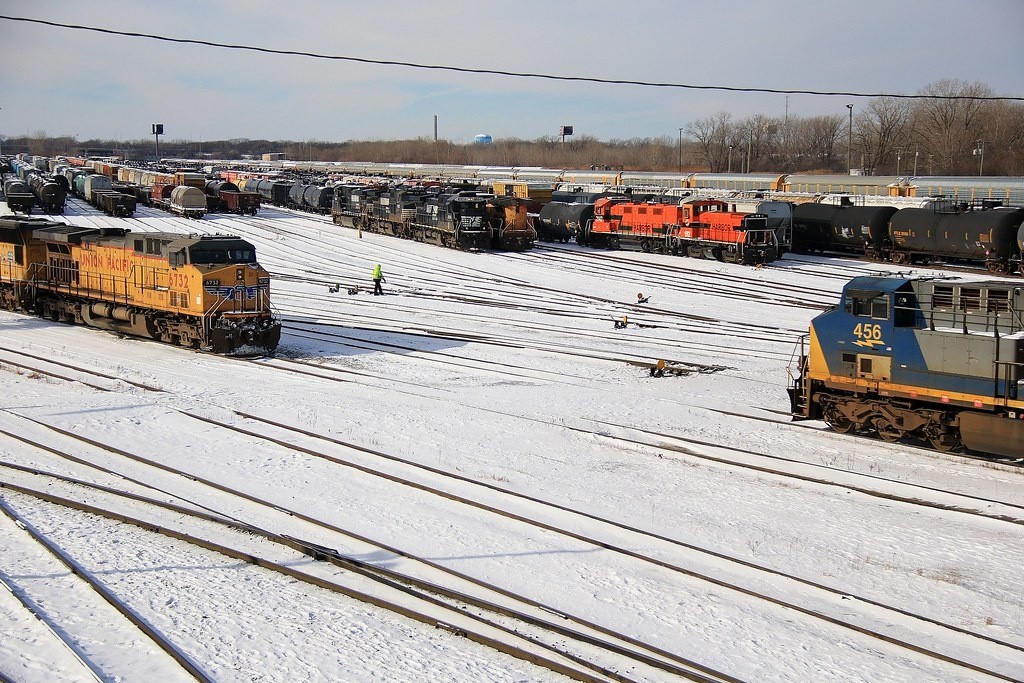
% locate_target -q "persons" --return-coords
[373,263,386,295]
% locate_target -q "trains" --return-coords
[0,214,280,364]
[0,141,1022,272]
[787,273,1024,467]
